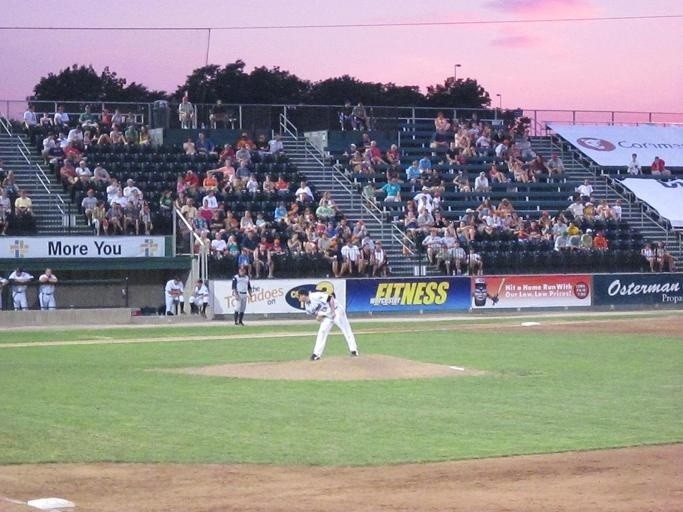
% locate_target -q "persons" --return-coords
[376,145,622,279]
[337,100,373,132]
[209,100,229,129]
[38,267,58,310]
[627,152,644,176]
[429,112,536,158]
[1,163,38,236]
[160,132,388,279]
[295,287,359,362]
[56,158,154,237]
[653,241,678,273]
[640,242,663,273]
[471,279,498,307]
[188,279,209,319]
[179,97,195,129]
[649,155,671,175]
[7,266,35,312]
[0,275,9,311]
[25,104,154,166]
[230,268,252,328]
[163,274,186,317]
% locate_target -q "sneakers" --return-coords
[309,354,321,361]
[234,322,244,326]
[351,350,360,359]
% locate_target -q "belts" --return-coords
[16,291,23,293]
[42,292,52,296]
[236,291,248,294]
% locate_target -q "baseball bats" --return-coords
[491,278,505,306]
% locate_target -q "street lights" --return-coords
[497,94,503,109]
[454,64,461,81]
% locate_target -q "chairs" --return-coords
[32,120,354,277]
[426,219,648,274]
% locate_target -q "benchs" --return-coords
[331,123,588,225]
